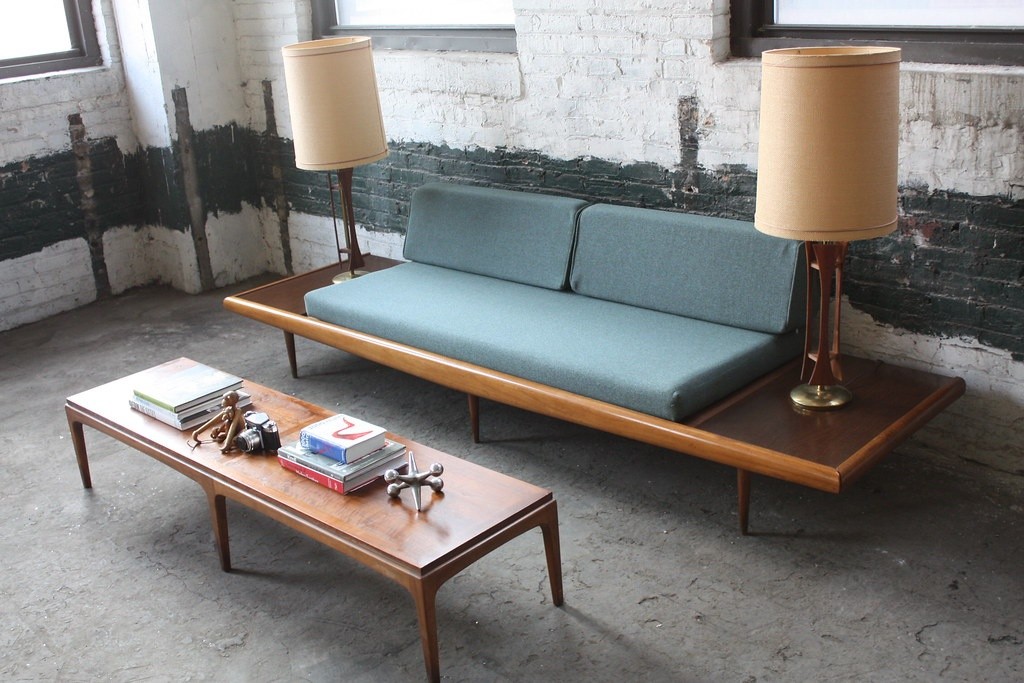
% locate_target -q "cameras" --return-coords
[237,410,282,453]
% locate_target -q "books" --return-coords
[277,412,409,496]
[126,358,254,432]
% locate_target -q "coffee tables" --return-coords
[64,356,565,683]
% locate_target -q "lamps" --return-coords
[281,36,392,284]
[752,45,902,412]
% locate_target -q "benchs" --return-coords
[222,182,968,537]
